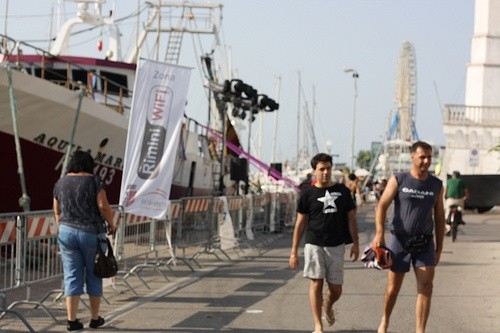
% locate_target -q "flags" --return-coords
[117,58,193,221]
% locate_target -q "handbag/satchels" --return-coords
[100,238,119,278]
[394,225,434,254]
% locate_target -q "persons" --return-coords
[371,142,446,333]
[53,151,116,331]
[443,171,469,237]
[298,174,314,194]
[288,153,360,333]
[341,174,388,209]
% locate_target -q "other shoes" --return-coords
[67,320,86,331]
[322,299,335,327]
[89,317,106,329]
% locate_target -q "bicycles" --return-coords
[447,204,464,243]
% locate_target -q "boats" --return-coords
[1,0,247,220]
